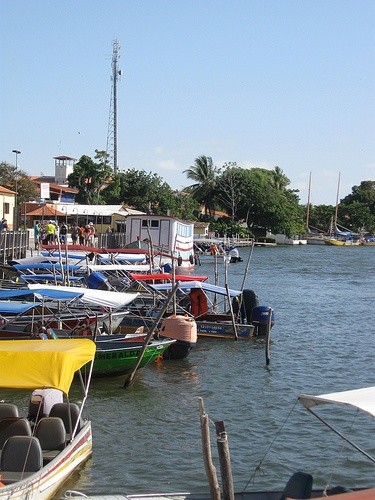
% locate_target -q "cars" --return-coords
[365,236,375,243]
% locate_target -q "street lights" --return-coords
[93,211,97,229]
[83,208,88,225]
[73,208,78,225]
[62,206,68,225]
[12,150,21,260]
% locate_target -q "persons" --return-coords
[59,222,68,244]
[105,226,113,234]
[69,221,95,248]
[34,221,59,250]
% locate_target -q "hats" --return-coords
[86,225,90,229]
[89,222,93,225]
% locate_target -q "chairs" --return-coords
[0,436,43,484]
[49,403,83,441]
[0,418,32,450]
[0,402,18,417]
[35,417,66,460]
[38,327,58,340]
[280,472,313,500]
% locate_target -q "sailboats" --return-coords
[306,170,360,246]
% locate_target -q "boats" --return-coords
[0,219,275,377]
[59,385,375,499]
[0,338,97,500]
